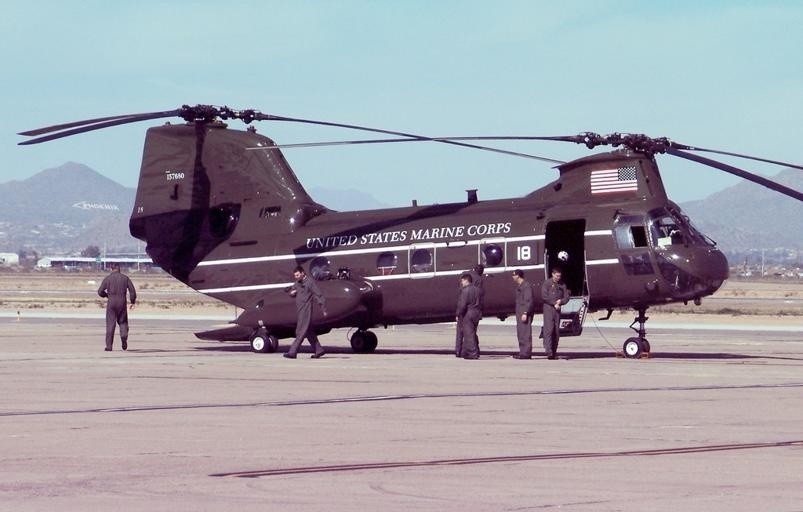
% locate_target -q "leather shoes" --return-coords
[513,353,531,359]
[121,336,127,350]
[283,354,296,358]
[311,351,326,358]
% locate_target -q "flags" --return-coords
[590,166,639,195]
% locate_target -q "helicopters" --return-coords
[16,105,803,358]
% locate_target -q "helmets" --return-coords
[557,250,568,261]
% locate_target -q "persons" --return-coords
[284,266,328,358]
[456,265,485,357]
[98,263,136,351]
[455,274,482,359]
[512,270,534,359]
[541,267,570,360]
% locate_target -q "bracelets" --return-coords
[523,313,527,315]
